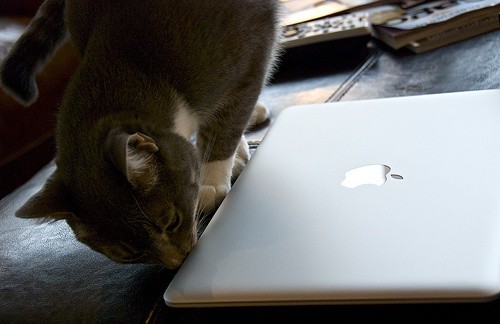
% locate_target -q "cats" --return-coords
[1,0,286,271]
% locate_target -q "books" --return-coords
[343,1,500,54]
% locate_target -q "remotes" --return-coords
[275,10,369,48]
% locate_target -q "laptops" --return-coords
[157,86,500,301]
